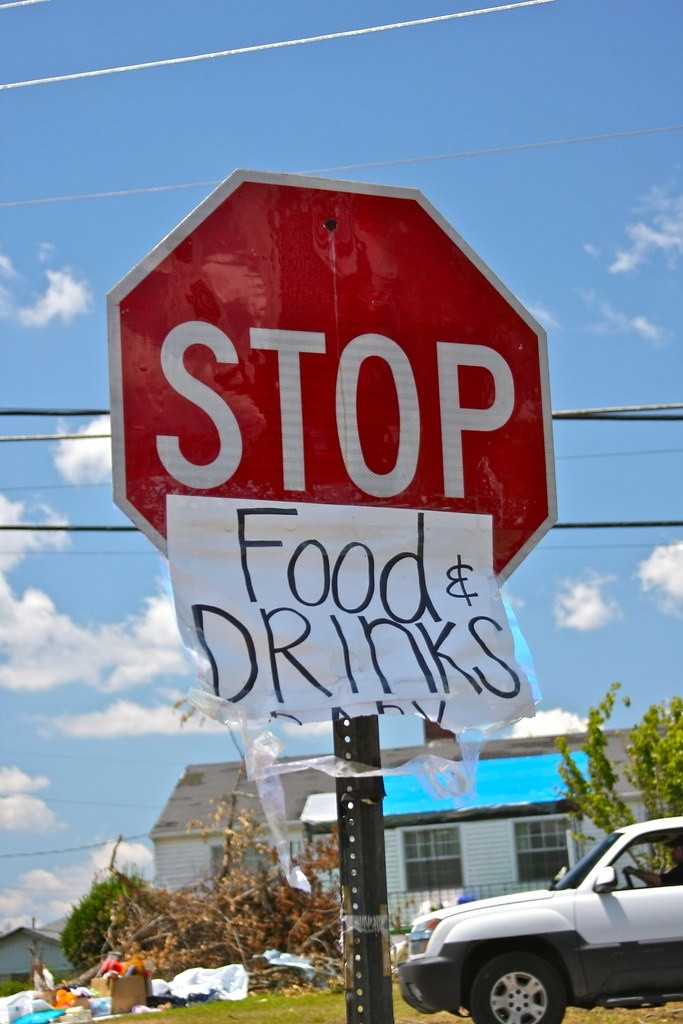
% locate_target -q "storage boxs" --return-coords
[88,977,147,1015]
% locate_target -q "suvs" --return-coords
[396,815,683,1023]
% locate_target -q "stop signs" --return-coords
[104,167,557,587]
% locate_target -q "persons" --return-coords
[622,835,683,887]
